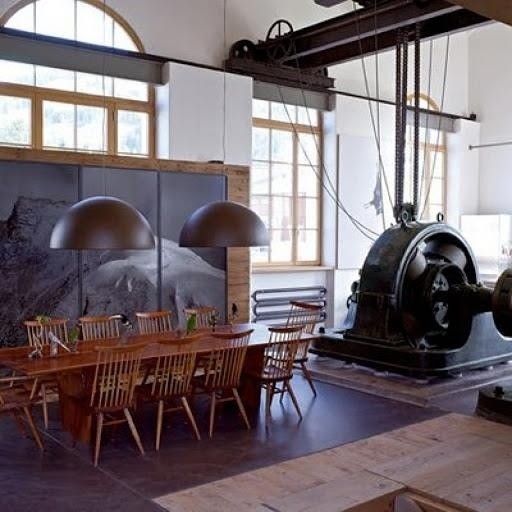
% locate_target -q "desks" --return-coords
[1,323,315,442]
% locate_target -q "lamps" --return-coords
[48,0,156,253]
[176,1,274,249]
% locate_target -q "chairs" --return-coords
[71,341,146,468]
[138,332,202,451]
[263,301,315,396]
[1,374,43,448]
[22,319,70,428]
[244,324,303,429]
[192,329,252,438]
[79,307,219,397]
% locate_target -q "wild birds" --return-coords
[232,302,239,320]
[209,309,218,332]
[68,327,81,344]
[182,309,197,336]
[34,315,51,327]
[48,331,70,353]
[108,313,132,347]
